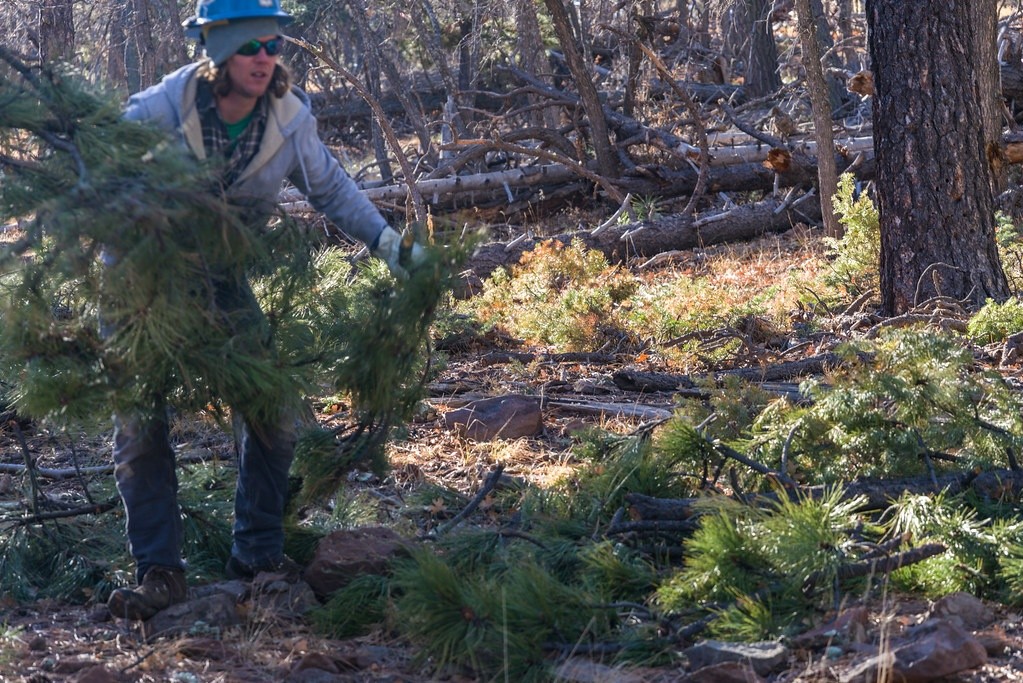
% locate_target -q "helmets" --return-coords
[184,1,297,27]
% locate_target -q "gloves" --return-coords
[371,229,425,284]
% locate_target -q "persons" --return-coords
[108,0,429,622]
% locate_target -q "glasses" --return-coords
[237,37,282,56]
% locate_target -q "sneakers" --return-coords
[224,557,252,580]
[108,567,187,620]
